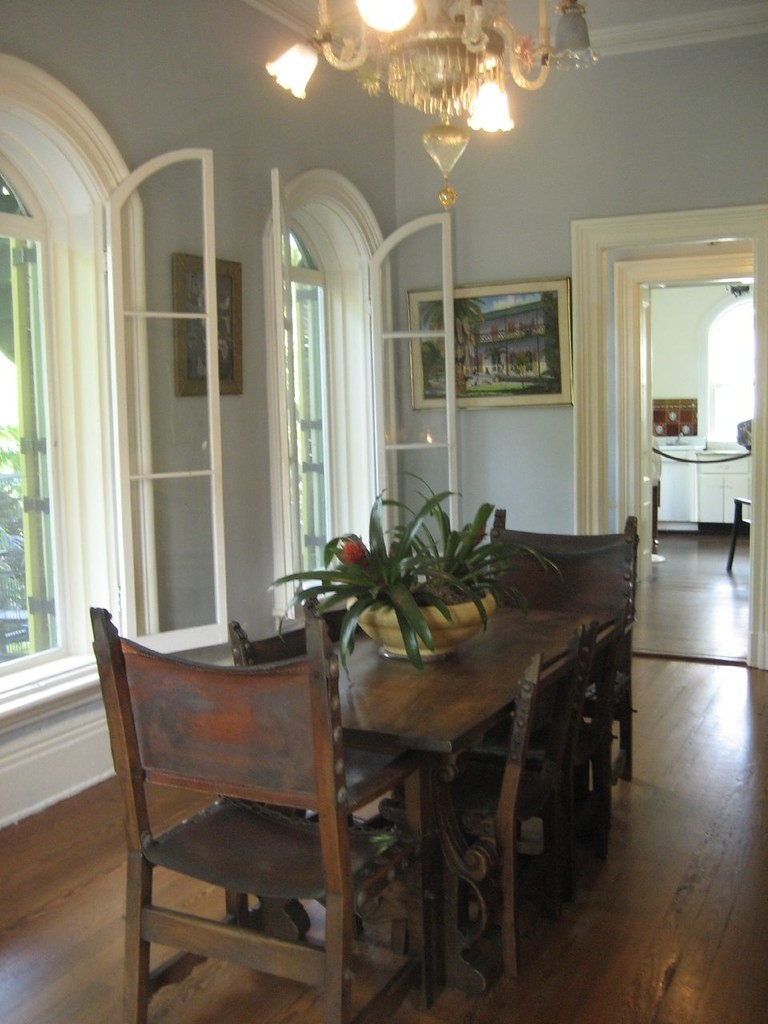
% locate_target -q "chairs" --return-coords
[231,509,642,983]
[88,603,433,1024]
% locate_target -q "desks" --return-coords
[244,603,627,934]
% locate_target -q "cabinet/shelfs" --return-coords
[698,455,753,525]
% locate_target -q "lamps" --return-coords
[264,0,601,208]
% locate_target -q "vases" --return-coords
[344,589,498,661]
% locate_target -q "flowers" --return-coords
[264,470,567,672]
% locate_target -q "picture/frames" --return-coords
[403,279,575,410]
[171,250,243,396]
